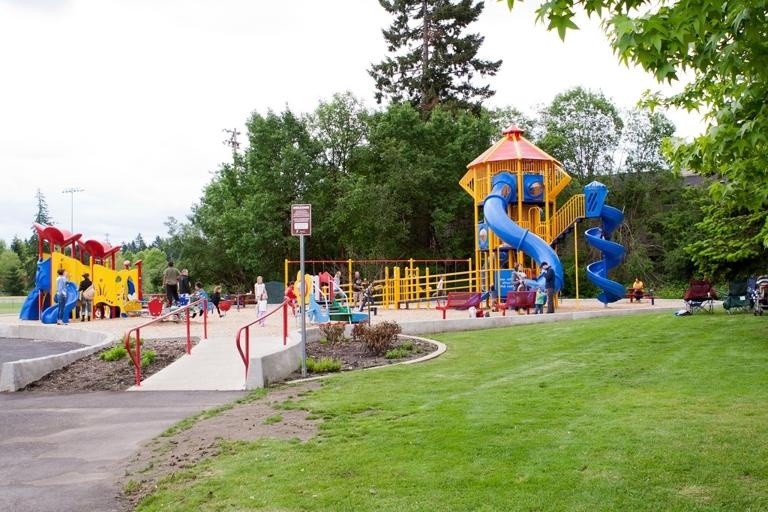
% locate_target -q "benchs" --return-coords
[432,291,486,320]
[625,287,658,305]
[494,287,537,315]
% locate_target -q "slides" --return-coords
[585,205,626,304]
[41,282,78,323]
[456,292,489,309]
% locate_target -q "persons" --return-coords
[514,269,526,291]
[77,273,93,322]
[745,272,757,311]
[535,261,556,313]
[332,271,345,306]
[189,281,207,318]
[161,261,181,308]
[255,292,267,327]
[532,286,547,314]
[432,275,446,306]
[284,281,298,316]
[352,271,366,312]
[56,268,68,326]
[209,285,224,318]
[511,263,528,315]
[359,278,374,311]
[253,276,268,319]
[177,268,192,295]
[632,277,643,302]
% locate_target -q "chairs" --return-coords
[722,273,755,317]
[682,279,719,317]
